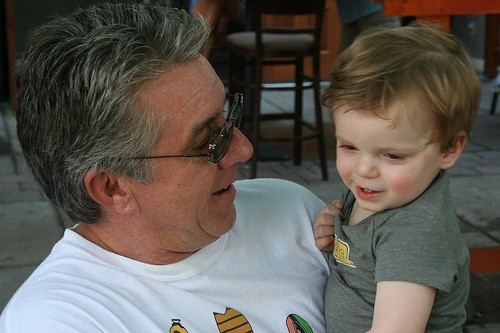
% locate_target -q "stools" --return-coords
[225,0,330,180]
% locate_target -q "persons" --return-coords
[1,1,331,333]
[312,22,482,333]
[188,0,241,69]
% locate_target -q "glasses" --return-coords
[107,92,244,164]
